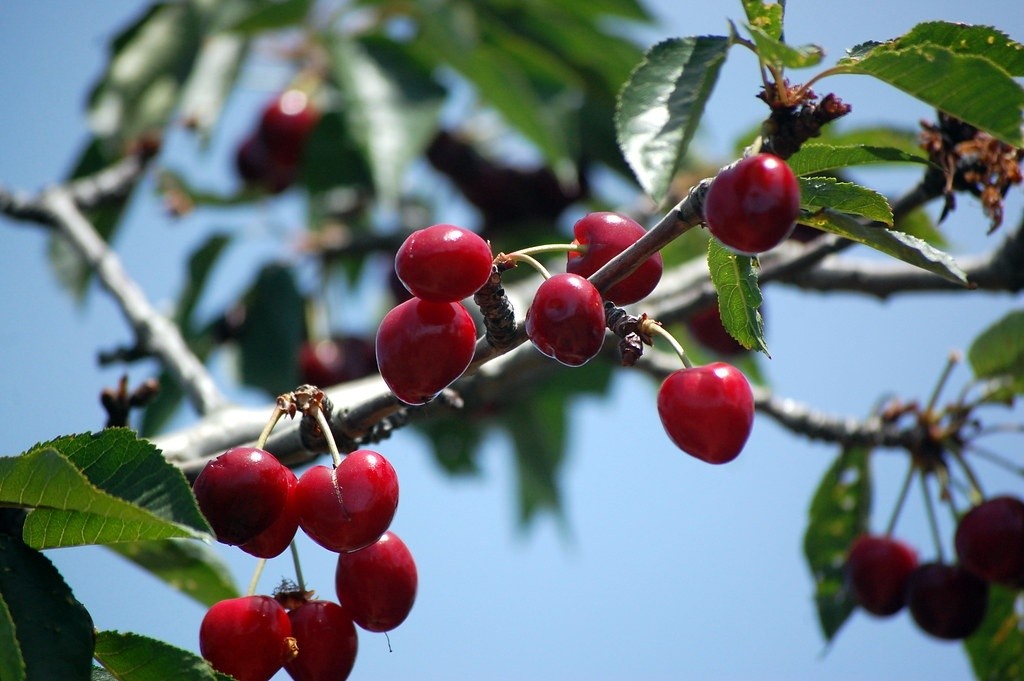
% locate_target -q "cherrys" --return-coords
[187,95,1024,681]
